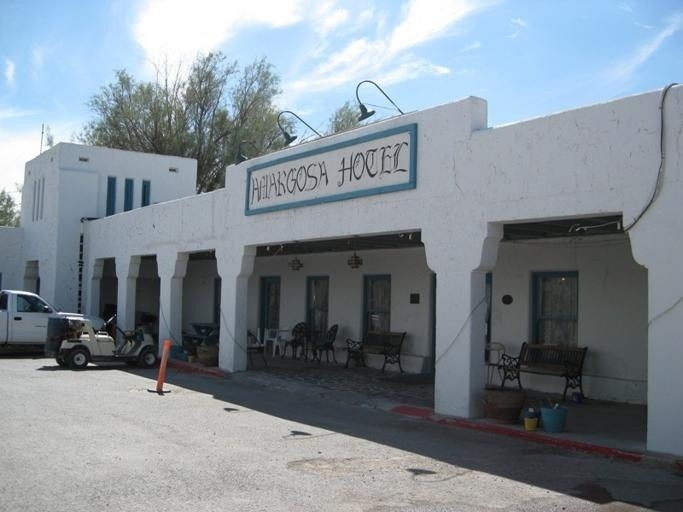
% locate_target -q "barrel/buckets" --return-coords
[540,405,568,433]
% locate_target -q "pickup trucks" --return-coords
[0,289,106,359]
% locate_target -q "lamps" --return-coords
[235,139,267,166]
[288,241,304,270]
[277,110,324,147]
[355,79,405,121]
[347,235,362,269]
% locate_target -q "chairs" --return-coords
[311,324,338,364]
[247,331,267,368]
[281,322,311,361]
[484,342,506,390]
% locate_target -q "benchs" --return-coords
[345,331,408,373]
[497,341,589,403]
